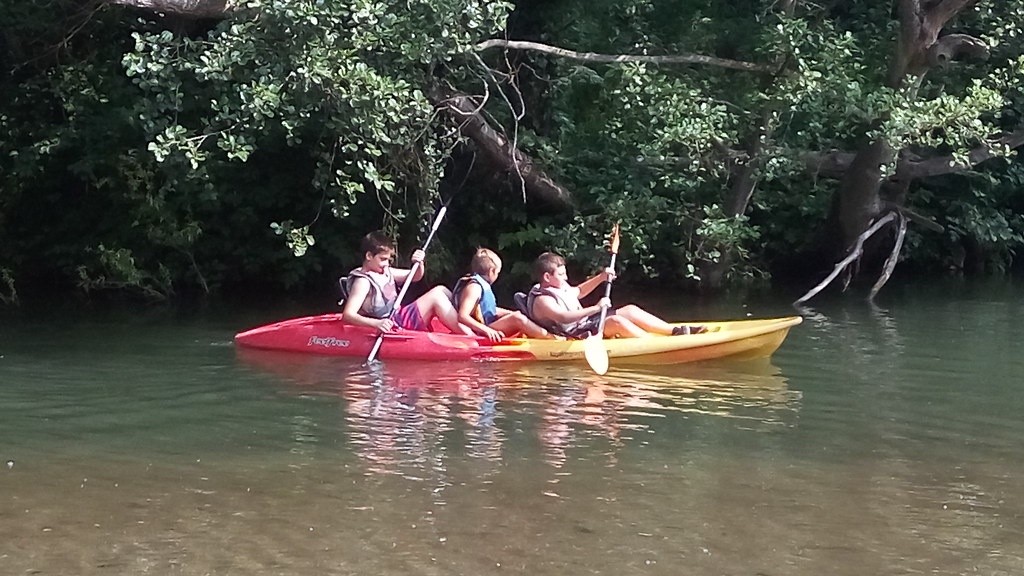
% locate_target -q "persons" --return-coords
[343,230,476,336]
[451,248,591,343]
[527,252,707,339]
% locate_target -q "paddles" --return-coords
[361,206,447,368]
[584,223,620,376]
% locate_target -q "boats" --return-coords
[233,316,803,363]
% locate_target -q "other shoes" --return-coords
[673,324,690,336]
[690,326,706,334]
